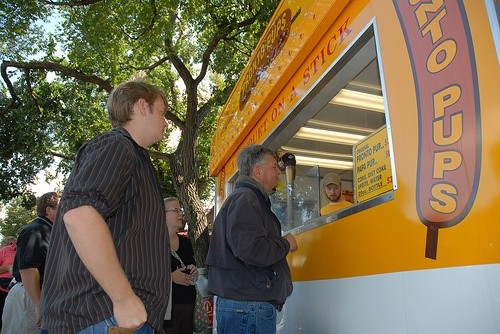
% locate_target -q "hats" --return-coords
[322,172,341,188]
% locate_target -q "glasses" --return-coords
[165,208,185,213]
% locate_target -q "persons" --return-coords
[0,236,18,331]
[162,197,199,334]
[204,144,299,334]
[319,172,353,215]
[1,192,61,334]
[41,81,172,334]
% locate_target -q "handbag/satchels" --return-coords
[195,293,209,332]
